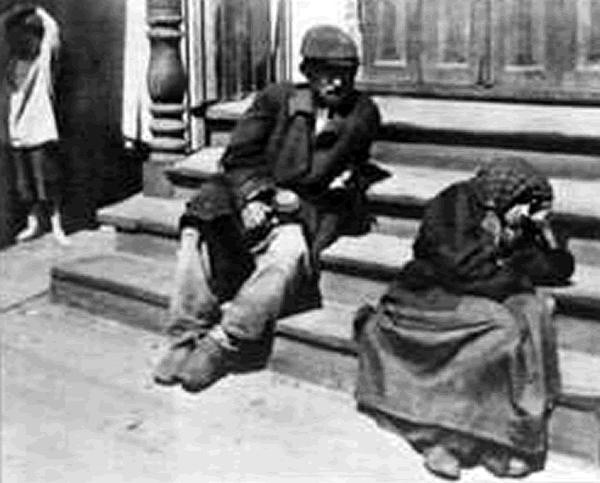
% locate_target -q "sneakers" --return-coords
[153,334,199,387]
[422,445,462,480]
[180,334,230,392]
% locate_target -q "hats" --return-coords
[301,25,359,68]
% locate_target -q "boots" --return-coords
[50,211,71,248]
[14,214,44,243]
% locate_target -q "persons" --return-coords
[1,1,71,242]
[352,154,579,481]
[152,23,383,393]
